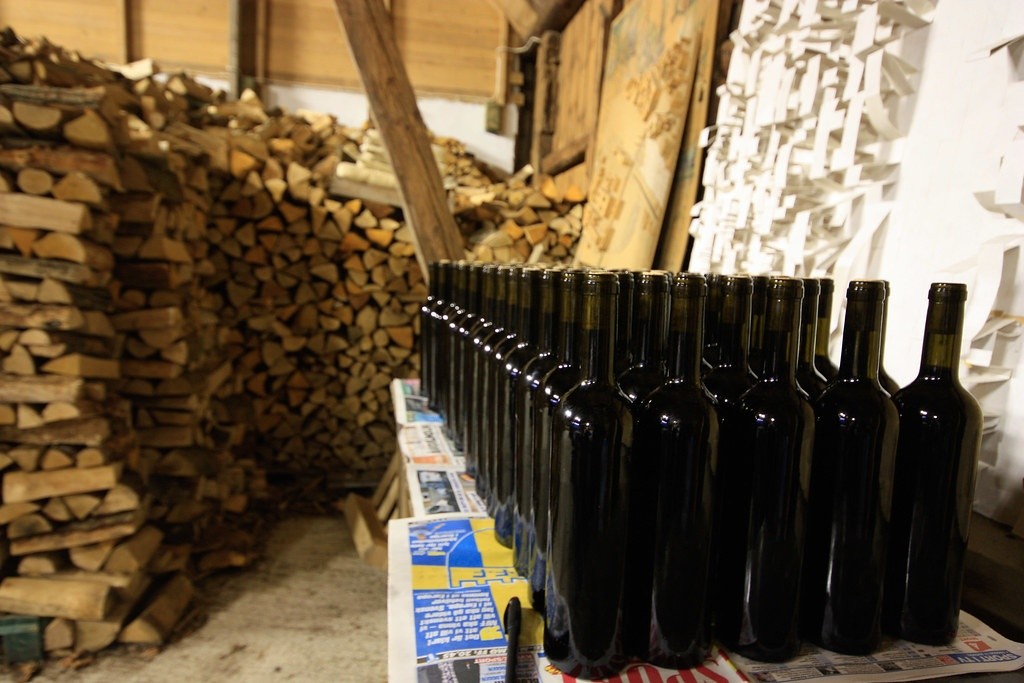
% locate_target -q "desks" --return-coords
[388,376,1024,683]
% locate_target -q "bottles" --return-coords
[416,258,986,682]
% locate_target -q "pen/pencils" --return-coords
[503,596,522,683]
